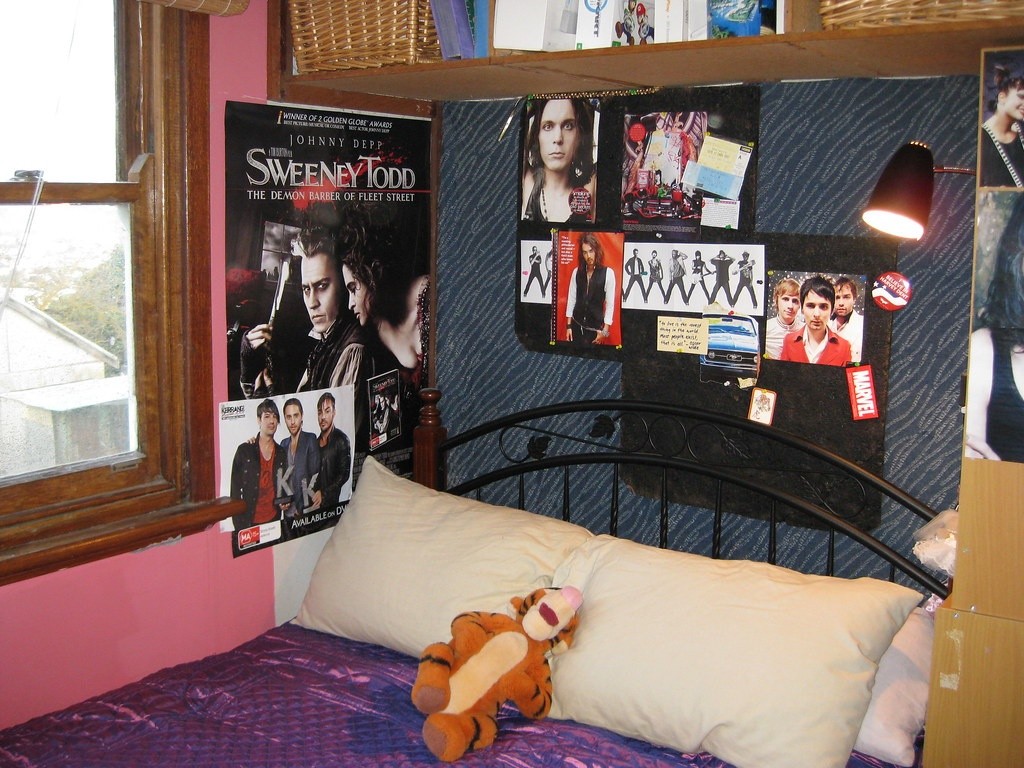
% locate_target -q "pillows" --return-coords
[290,454,935,768]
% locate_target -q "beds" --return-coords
[0,393,948,768]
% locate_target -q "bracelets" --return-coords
[566,324,572,330]
[601,329,610,338]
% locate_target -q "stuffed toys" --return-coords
[412,585,585,762]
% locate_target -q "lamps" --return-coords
[863,142,978,238]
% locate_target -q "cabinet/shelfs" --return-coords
[924,38,1024,768]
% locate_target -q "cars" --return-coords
[700,314,759,373]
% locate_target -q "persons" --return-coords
[373,395,399,434]
[233,392,351,528]
[341,227,430,395]
[520,244,546,300]
[238,226,384,453]
[623,247,763,312]
[564,231,615,346]
[967,193,1024,461]
[980,67,1024,187]
[520,98,599,228]
[764,273,864,366]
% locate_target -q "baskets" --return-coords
[288,0,448,76]
[820,1,1024,32]
